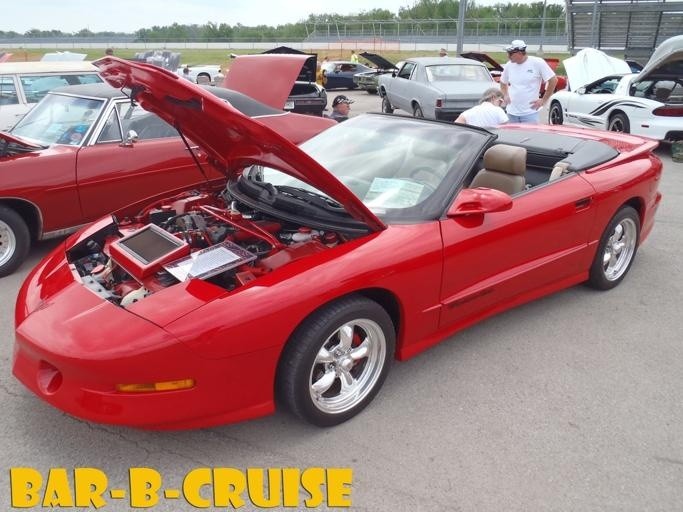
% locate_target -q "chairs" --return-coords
[396,138,458,188]
[467,142,528,198]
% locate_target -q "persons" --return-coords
[440,48,449,59]
[350,49,358,63]
[329,95,355,123]
[499,39,559,126]
[218,70,224,78]
[316,62,327,87]
[182,68,195,83]
[322,56,330,64]
[105,48,113,56]
[453,87,509,129]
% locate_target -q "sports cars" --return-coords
[12,54,663,430]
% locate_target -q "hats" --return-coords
[440,47,448,54]
[502,39,527,52]
[332,95,355,107]
